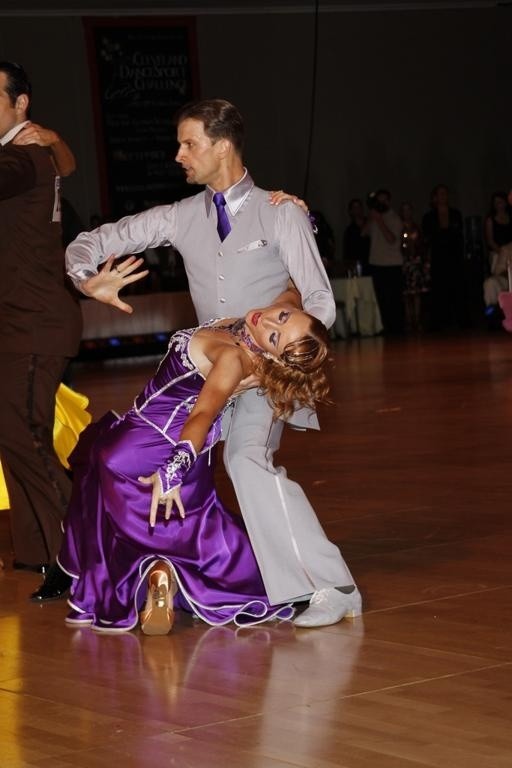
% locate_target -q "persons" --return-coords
[27,96,364,633]
[309,181,511,337]
[27,188,340,636]
[0,54,83,575]
[1,108,92,518]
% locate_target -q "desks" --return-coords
[79,277,384,362]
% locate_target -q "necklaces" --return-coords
[205,316,264,359]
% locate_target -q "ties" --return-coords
[212,191,232,245]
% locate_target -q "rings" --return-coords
[114,265,122,273]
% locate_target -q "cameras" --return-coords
[366,192,388,212]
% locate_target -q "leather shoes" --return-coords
[288,587,362,630]
[30,559,73,605]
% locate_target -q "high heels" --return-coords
[137,560,178,638]
